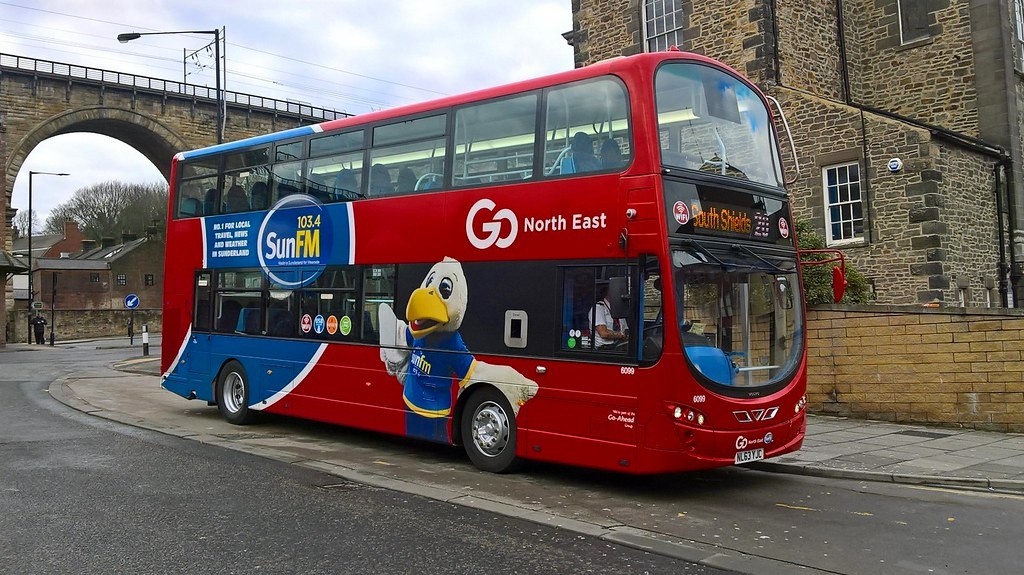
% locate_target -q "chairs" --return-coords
[195,297,376,342]
[180,132,623,217]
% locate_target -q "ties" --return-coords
[613,318,621,332]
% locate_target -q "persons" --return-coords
[125,317,132,337]
[30,312,48,345]
[588,286,630,353]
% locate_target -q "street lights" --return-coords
[117,22,228,142]
[25,170,71,344]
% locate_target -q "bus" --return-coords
[162,53,849,477]
